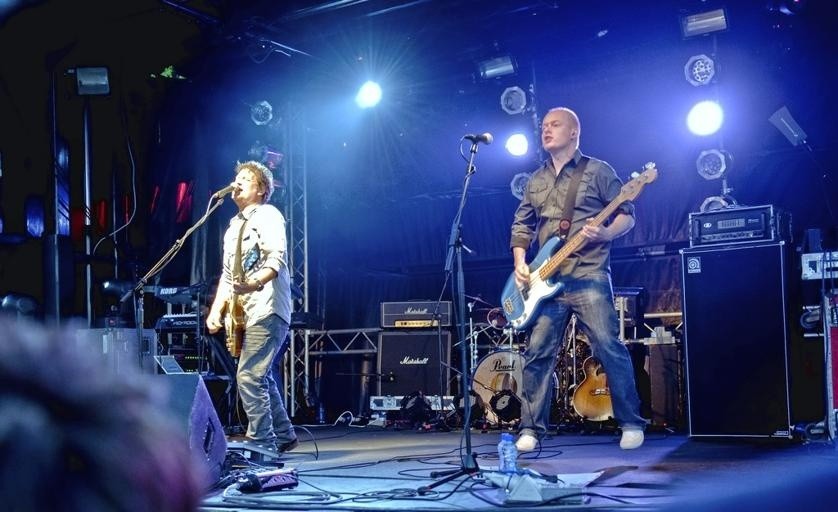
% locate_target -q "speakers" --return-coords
[145,372,227,490]
[680,244,793,444]
[377,332,452,399]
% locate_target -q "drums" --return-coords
[470,349,559,426]
[569,328,592,361]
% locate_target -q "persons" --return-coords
[206,160,298,457]
[510,106,648,452]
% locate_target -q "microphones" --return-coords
[212,181,239,200]
[466,133,493,146]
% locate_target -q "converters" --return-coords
[238,468,300,492]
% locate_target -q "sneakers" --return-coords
[619,429,644,450]
[515,433,538,451]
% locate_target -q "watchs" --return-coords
[253,278,264,292]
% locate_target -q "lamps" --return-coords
[77,65,111,96]
[248,98,282,128]
[684,53,717,89]
[699,194,735,213]
[498,85,533,118]
[509,171,531,203]
[475,51,518,82]
[678,5,729,41]
[695,147,733,182]
[766,105,836,212]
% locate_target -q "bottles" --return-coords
[498,433,520,475]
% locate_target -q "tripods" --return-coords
[418,145,558,493]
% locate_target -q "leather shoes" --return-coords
[277,437,300,453]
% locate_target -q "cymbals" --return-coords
[479,307,494,310]
[463,293,492,307]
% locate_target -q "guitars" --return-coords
[572,344,615,422]
[222,209,256,360]
[499,162,658,330]
